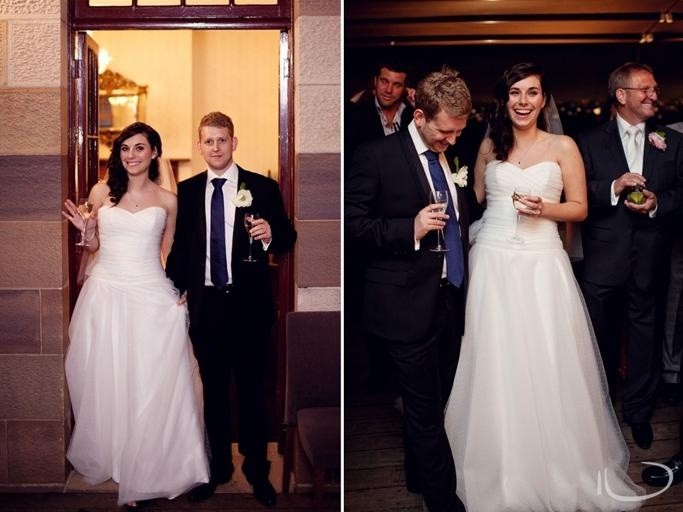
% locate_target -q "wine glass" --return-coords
[242,213,261,262]
[512,188,531,240]
[75,197,96,246]
[429,190,451,252]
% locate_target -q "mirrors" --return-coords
[96,68,150,157]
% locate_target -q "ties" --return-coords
[210,178,228,289]
[424,151,464,289]
[626,127,640,174]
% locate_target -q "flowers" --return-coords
[451,156,468,188]
[648,131,667,151]
[232,184,255,207]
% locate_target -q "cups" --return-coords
[626,181,646,204]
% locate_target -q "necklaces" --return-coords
[135,205,138,208]
[518,161,522,165]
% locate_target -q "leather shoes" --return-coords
[188,474,231,501]
[424,493,464,512]
[253,481,276,506]
[632,424,653,450]
[642,453,682,486]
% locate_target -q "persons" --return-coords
[444,64,648,512]
[165,113,297,510]
[345,61,412,211]
[345,66,482,511]
[61,123,211,511]
[576,61,683,451]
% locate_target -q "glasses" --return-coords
[622,86,659,97]
